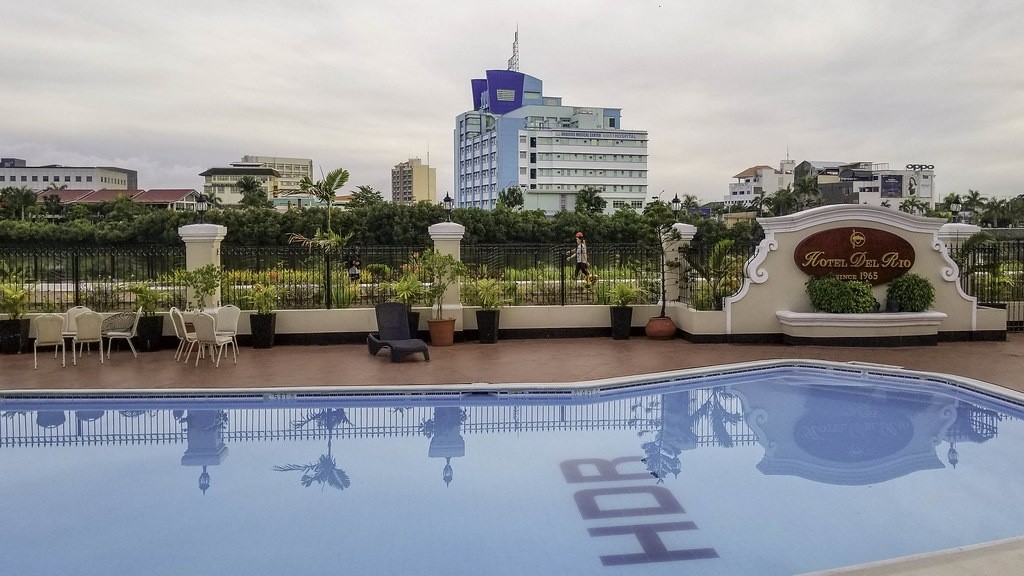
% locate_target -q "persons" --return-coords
[566,232,590,276]
[348,242,362,292]
[567,236,590,281]
[906,177,917,198]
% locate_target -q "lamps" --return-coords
[670,453,680,479]
[442,457,453,488]
[947,442,958,469]
[950,194,963,225]
[670,193,683,224]
[442,191,454,222]
[198,466,209,495]
[195,191,209,223]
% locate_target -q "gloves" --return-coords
[567,256,572,261]
[586,262,590,267]
[566,251,570,255]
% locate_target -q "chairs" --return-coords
[366,302,430,363]
[33,312,65,370]
[192,312,236,369]
[106,305,142,358]
[36,411,65,429]
[215,305,240,359]
[74,411,103,422]
[54,306,92,359]
[169,307,202,362]
[72,311,104,366]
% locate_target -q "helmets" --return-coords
[576,232,583,237]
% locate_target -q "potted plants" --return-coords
[174,262,223,353]
[125,274,166,352]
[417,248,468,347]
[0,285,35,355]
[385,273,426,339]
[604,282,636,340]
[977,266,1015,310]
[239,276,288,350]
[635,199,686,339]
[466,276,514,344]
